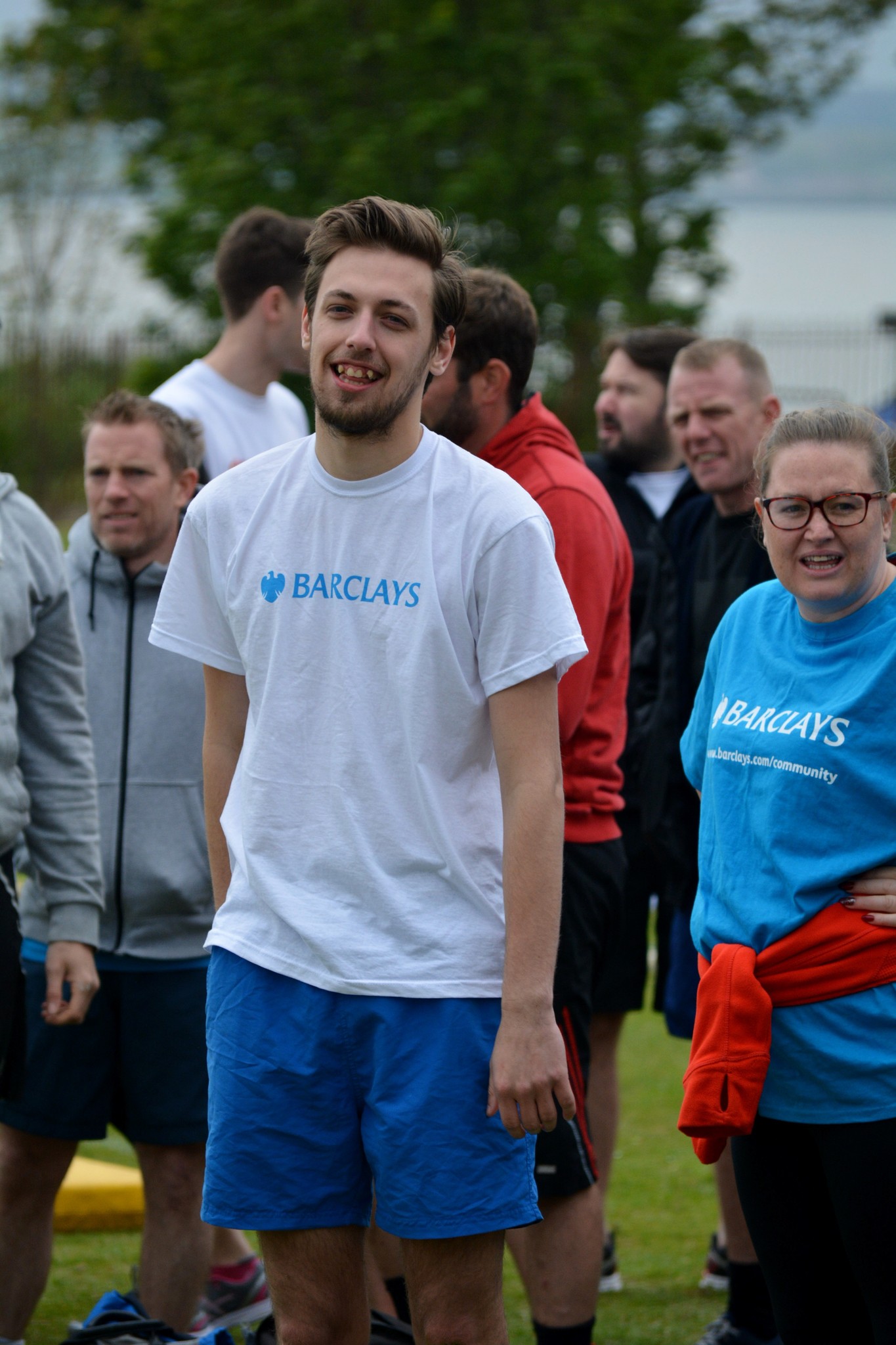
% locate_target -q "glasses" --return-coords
[759,491,893,530]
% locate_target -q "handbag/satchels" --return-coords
[254,1303,417,1345]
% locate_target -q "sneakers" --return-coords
[60,1265,235,1345]
[187,1254,272,1338]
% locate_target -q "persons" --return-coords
[578,324,730,1293]
[419,264,635,1345]
[627,338,782,1345]
[147,207,314,1338]
[147,196,590,1345]
[0,472,106,1237]
[0,386,236,1345]
[678,397,896,1345]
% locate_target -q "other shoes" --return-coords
[698,1234,731,1289]
[696,1307,783,1345]
[600,1230,624,1293]
